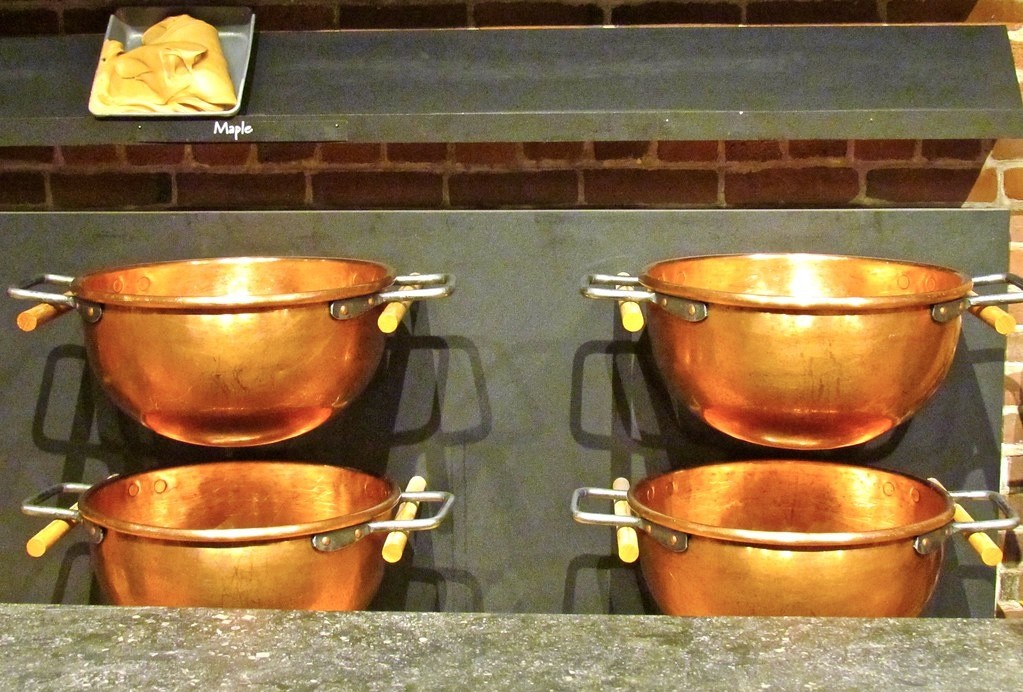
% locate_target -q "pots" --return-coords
[578,250,1023,451]
[5,254,457,447]
[20,459,453,611]
[568,459,1020,616]
